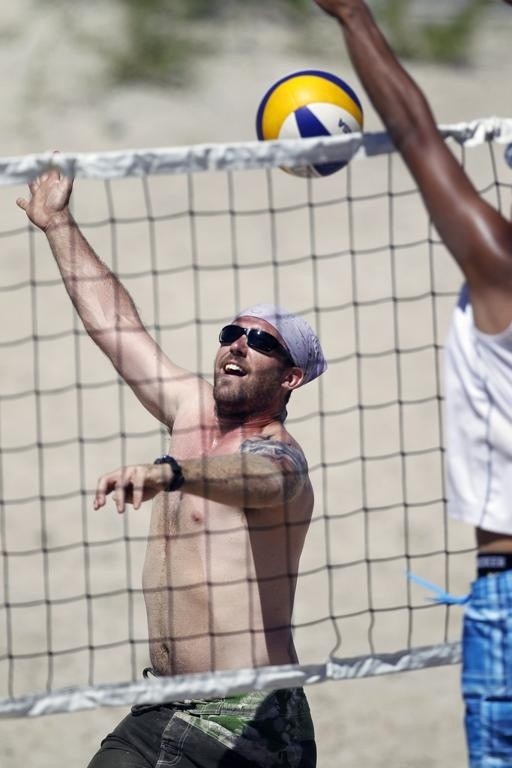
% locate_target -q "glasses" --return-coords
[219,324,289,364]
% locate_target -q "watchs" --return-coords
[154,456,184,493]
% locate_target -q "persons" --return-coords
[312,0,512,768]
[15,148,326,768]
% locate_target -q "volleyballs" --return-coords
[257,70,363,179]
